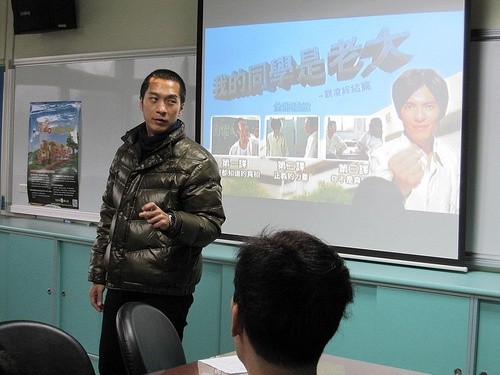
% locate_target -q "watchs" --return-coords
[167,213,175,230]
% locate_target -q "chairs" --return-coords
[0,319,94,375]
[116,300,187,374]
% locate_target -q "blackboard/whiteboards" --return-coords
[11,37,500,258]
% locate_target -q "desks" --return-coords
[153,348,426,374]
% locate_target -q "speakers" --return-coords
[11,0,77,35]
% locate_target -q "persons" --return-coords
[302,117,318,158]
[357,118,383,160]
[87,68,226,375]
[266,118,289,157]
[228,118,259,157]
[326,120,347,159]
[370,69,461,214]
[231,229,355,375]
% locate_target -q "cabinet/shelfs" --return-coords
[0,210,498,375]
